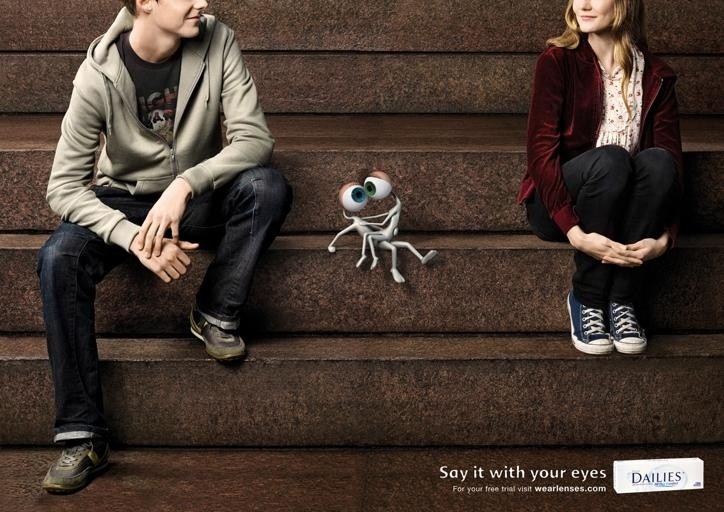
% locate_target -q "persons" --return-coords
[517,0,682,354]
[39,0,293,496]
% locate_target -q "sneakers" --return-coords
[189,303,247,361]
[41,436,110,495]
[607,296,648,354]
[566,286,614,355]
